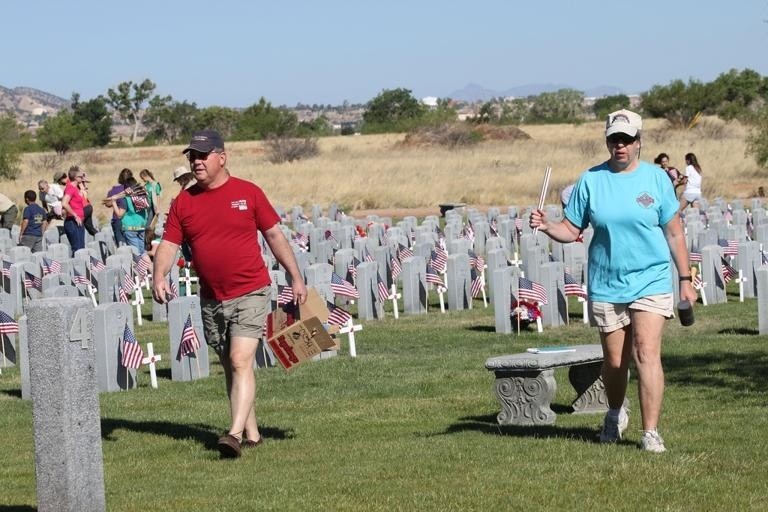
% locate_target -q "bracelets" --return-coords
[527,110,697,453]
[678,276,692,282]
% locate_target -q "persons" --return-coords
[153,131,310,457]
[674,153,702,211]
[1,164,194,263]
[654,153,680,192]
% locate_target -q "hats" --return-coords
[183,132,224,153]
[173,167,192,180]
[606,108,642,138]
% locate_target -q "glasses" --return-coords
[39,188,44,191]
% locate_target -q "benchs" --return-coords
[486,344,632,428]
[439,203,466,217]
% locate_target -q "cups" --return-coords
[676,300,694,326]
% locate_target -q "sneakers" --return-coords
[241,436,262,448]
[600,409,628,442]
[218,434,241,457]
[641,432,666,452]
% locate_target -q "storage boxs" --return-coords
[266,286,340,372]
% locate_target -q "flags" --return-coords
[467,249,484,298]
[489,216,523,240]
[0,249,208,389]
[278,285,295,304]
[519,277,548,304]
[279,211,309,252]
[325,211,448,303]
[327,301,351,329]
[565,268,588,300]
[458,220,474,241]
[673,199,767,304]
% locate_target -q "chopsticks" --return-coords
[533,166,552,237]
